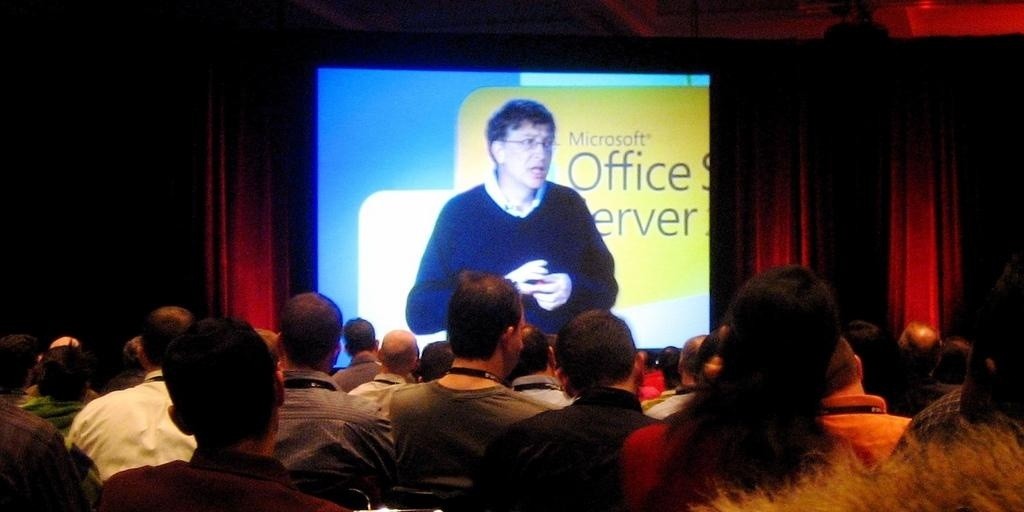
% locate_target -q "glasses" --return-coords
[499,135,557,152]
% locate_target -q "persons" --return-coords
[494,309,664,512]
[273,293,395,479]
[20,346,90,438]
[99,337,148,394]
[505,326,573,407]
[661,346,683,390]
[645,335,706,419]
[0,335,37,408]
[66,306,197,482]
[816,338,912,455]
[347,329,418,394]
[406,100,619,336]
[98,318,350,512]
[620,265,841,510]
[382,272,564,484]
[898,322,942,384]
[332,317,382,392]
